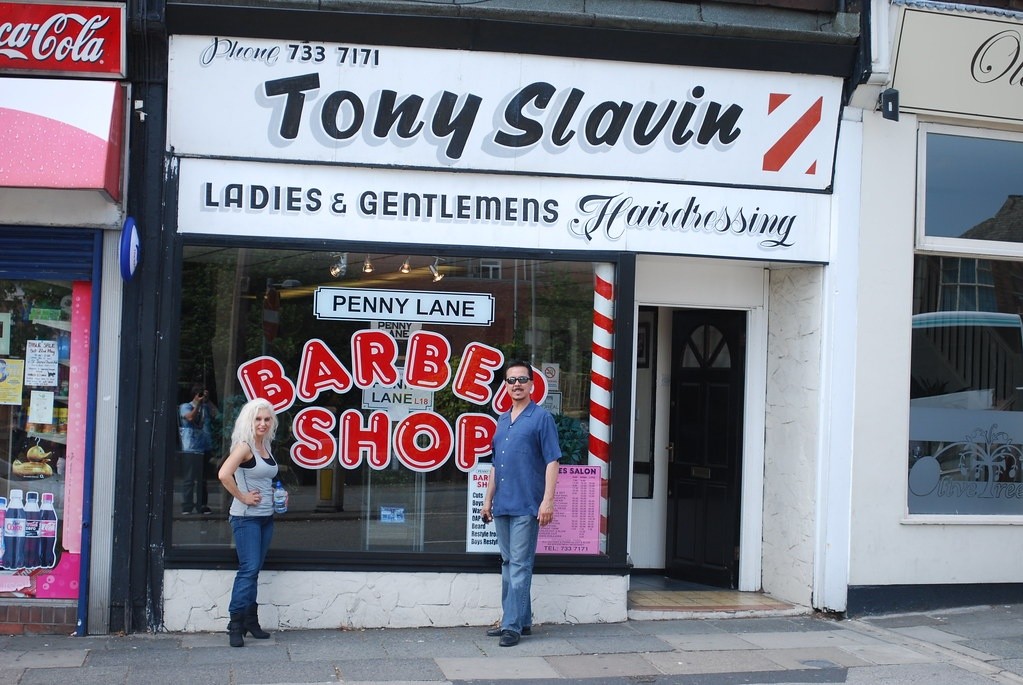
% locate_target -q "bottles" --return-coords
[4,489,26,568]
[274,482,287,513]
[40,493,56,568]
[0,497,8,566]
[23,493,40,568]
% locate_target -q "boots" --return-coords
[244,602,270,639]
[227,613,244,647]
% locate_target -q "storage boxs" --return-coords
[28,315,61,320]
[43,408,57,434]
[58,408,67,434]
[61,337,70,360]
[24,407,40,432]
[30,307,61,315]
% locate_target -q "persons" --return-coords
[219,398,287,648]
[481,361,562,647]
[178,386,216,515]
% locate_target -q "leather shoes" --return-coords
[499,630,520,646]
[487,626,531,636]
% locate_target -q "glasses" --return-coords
[506,376,530,384]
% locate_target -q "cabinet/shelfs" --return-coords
[23,299,67,445]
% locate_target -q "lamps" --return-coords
[878,88,899,122]
[399,255,412,274]
[328,253,350,277]
[362,254,375,273]
[428,256,445,282]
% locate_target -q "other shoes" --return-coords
[198,508,212,514]
[182,511,192,515]
